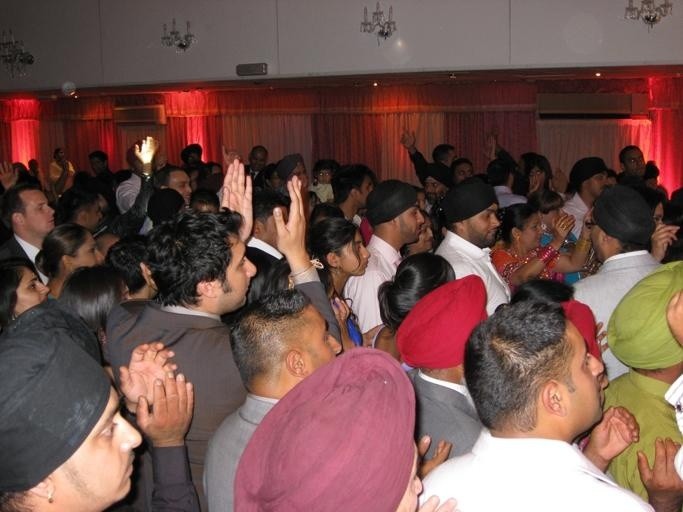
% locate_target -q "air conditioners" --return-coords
[112,106,164,123]
[536,92,631,116]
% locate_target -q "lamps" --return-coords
[0,27,36,80]
[360,1,397,47]
[160,19,198,55]
[621,0,673,35]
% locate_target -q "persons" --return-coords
[234,348,457,512]
[203,296,453,511]
[579,259,682,503]
[395,275,489,462]
[0,130,682,372]
[104,158,342,512]
[666,291,683,482]
[416,299,682,511]
[0,326,199,511]
